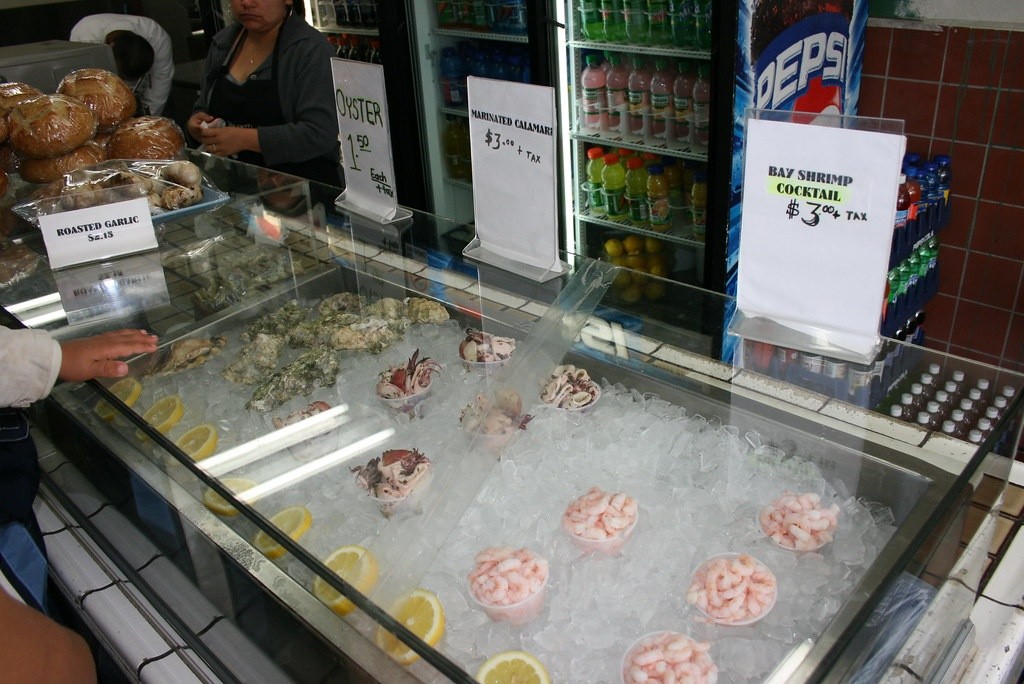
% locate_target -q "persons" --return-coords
[188,1,348,218]
[0,323,163,684]
[70,13,205,164]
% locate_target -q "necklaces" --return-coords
[250,58,256,64]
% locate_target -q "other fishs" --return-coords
[351,447,432,519]
[455,385,533,451]
[272,400,339,458]
[374,346,443,418]
[459,326,516,366]
[538,362,596,410]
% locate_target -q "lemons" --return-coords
[135,394,183,443]
[252,505,311,558]
[167,424,217,466]
[474,649,551,684]
[203,478,260,515]
[604,233,669,303]
[95,376,142,421]
[374,589,444,665]
[313,544,376,615]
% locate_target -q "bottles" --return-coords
[439,40,548,110]
[311,0,380,30]
[880,236,940,322]
[741,337,874,408]
[580,1,712,51]
[890,153,952,260]
[437,0,528,35]
[873,310,928,385]
[581,51,710,146]
[326,35,386,65]
[874,364,1014,446]
[442,114,473,183]
[587,144,706,235]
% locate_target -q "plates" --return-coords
[687,552,778,625]
[754,504,829,552]
[620,631,718,683]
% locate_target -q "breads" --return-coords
[0,67,203,240]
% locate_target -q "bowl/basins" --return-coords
[459,412,522,453]
[466,553,549,626]
[561,501,639,559]
[279,420,340,463]
[538,379,602,414]
[458,355,510,378]
[358,489,423,523]
[370,378,433,422]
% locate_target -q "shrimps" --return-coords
[759,490,839,550]
[686,554,772,623]
[624,632,719,684]
[562,487,635,553]
[468,545,547,618]
